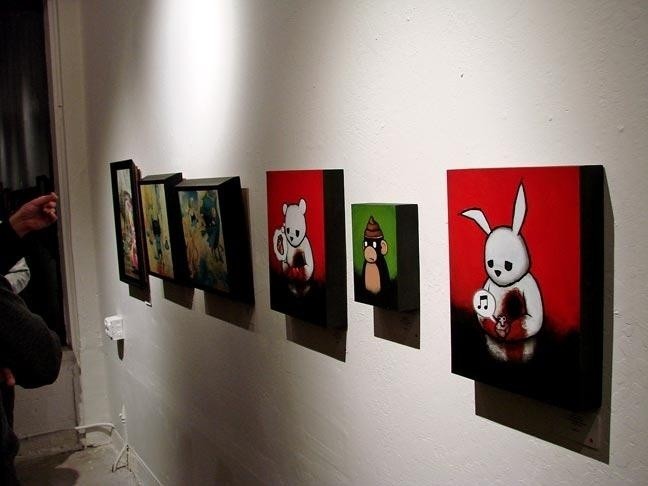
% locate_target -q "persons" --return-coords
[1,192,63,486]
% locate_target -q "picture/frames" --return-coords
[110,159,254,306]
